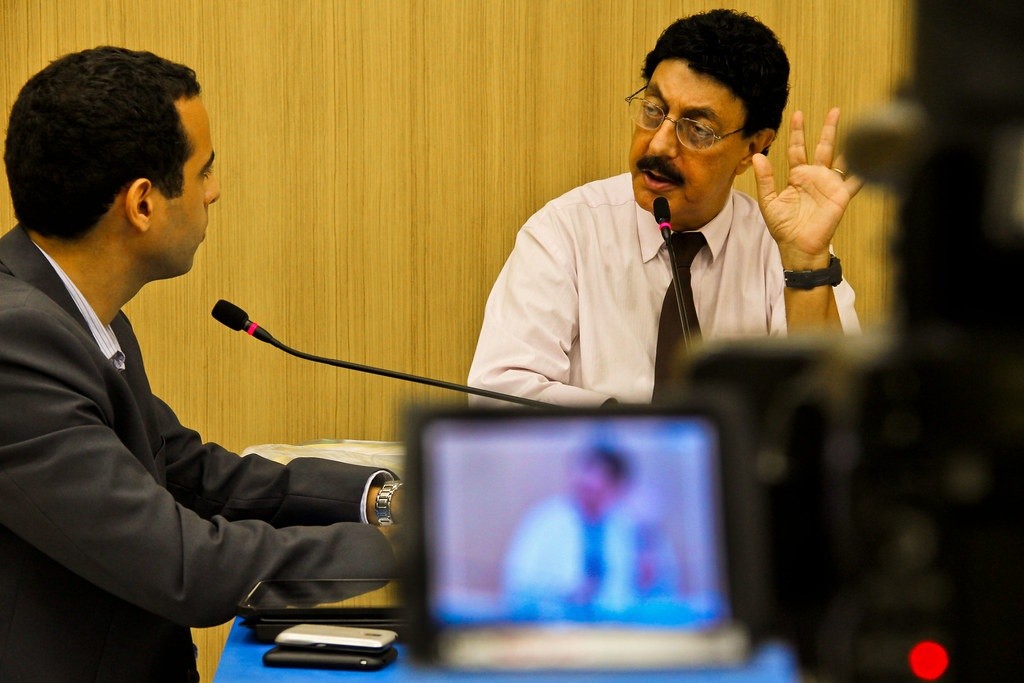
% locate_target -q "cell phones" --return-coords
[263,645,397,670]
[275,624,400,652]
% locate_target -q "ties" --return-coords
[651,232,707,405]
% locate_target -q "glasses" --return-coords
[626,85,746,152]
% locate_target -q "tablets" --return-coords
[233,577,409,621]
[404,399,747,663]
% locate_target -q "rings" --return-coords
[834,167,847,176]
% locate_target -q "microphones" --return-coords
[212,300,564,412]
[653,195,694,350]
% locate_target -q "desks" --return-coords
[210,613,802,683]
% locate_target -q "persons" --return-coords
[465,11,861,410]
[501,449,679,607]
[1,47,416,683]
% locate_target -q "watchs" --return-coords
[374,480,405,526]
[783,253,842,289]
